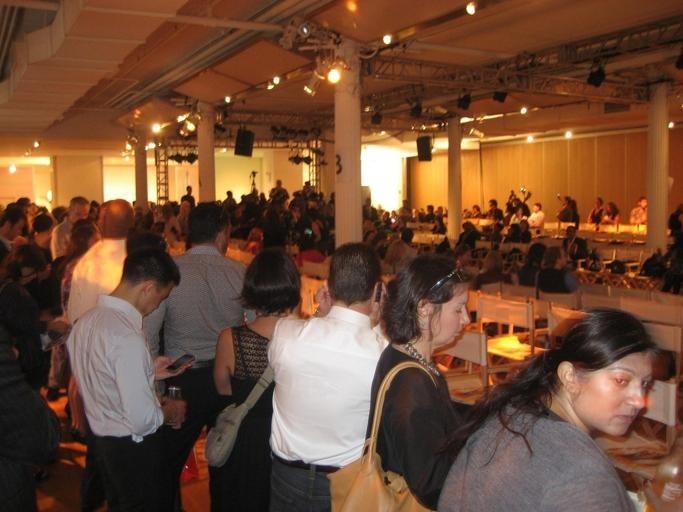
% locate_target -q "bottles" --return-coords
[645,433,683,512]
[165,386,183,429]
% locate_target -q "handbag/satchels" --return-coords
[205,403,248,468]
[326,453,434,512]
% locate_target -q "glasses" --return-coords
[430,267,466,290]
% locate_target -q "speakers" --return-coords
[234,129,255,156]
[417,136,432,162]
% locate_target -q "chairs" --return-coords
[402,214,671,278]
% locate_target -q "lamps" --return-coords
[327,63,343,84]
[304,69,325,98]
[186,112,202,131]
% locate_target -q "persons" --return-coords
[0,178,681,512]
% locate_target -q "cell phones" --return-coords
[165,353,195,374]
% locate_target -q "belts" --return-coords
[273,454,339,473]
